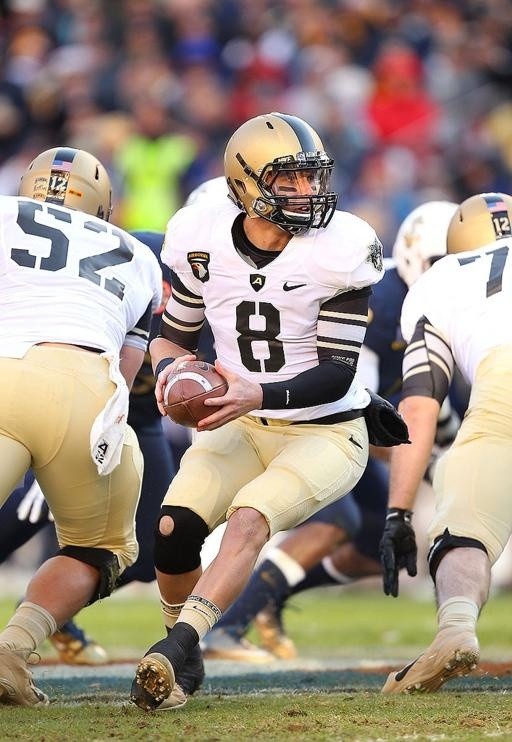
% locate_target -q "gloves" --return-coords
[376,505,421,599]
[363,385,413,449]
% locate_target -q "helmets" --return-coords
[17,144,117,225]
[221,109,335,221]
[445,191,512,256]
[392,199,461,292]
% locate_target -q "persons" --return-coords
[131,114,386,714]
[382,193,512,692]
[0,147,163,708]
[0,0,512,666]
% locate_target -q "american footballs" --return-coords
[162,361,229,427]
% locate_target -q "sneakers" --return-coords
[376,624,482,700]
[253,607,297,662]
[200,625,277,667]
[0,647,53,709]
[127,635,210,713]
[12,593,112,667]
